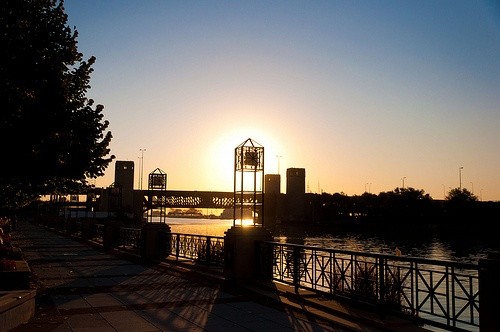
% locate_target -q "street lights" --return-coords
[459,167,463,192]
[140,149,146,190]
[403,177,406,188]
[137,156,142,189]
[364,182,368,193]
